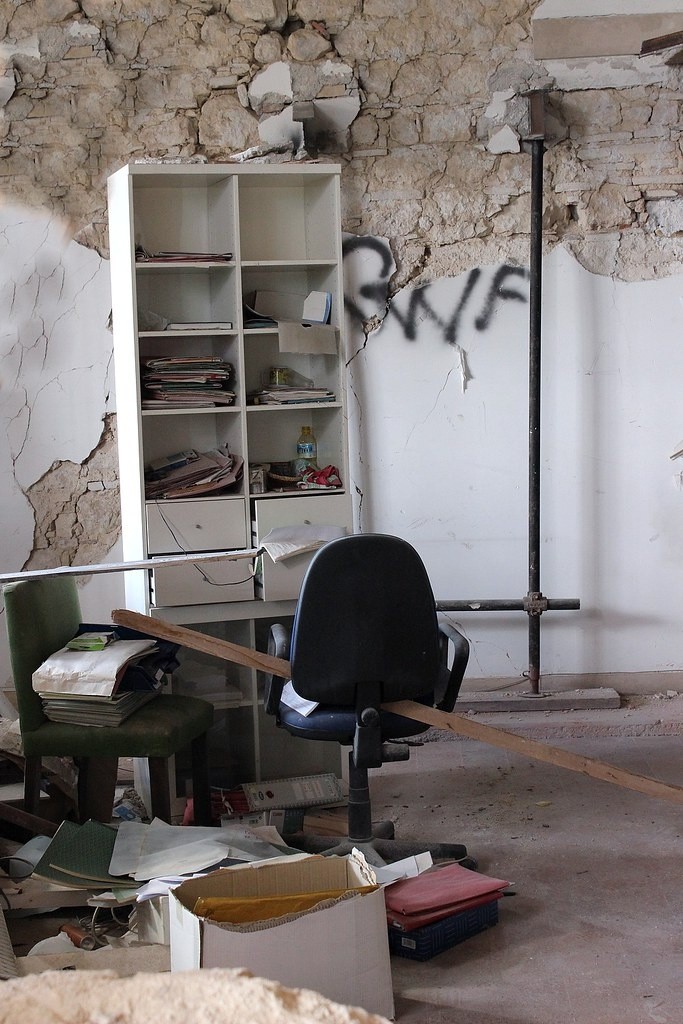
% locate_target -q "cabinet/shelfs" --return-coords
[104,161,362,788]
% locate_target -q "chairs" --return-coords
[3,563,219,829]
[263,532,478,872]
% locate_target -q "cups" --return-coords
[262,365,289,387]
[249,466,266,493]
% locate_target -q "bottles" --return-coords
[297,425,317,469]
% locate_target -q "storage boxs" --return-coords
[166,844,436,1020]
